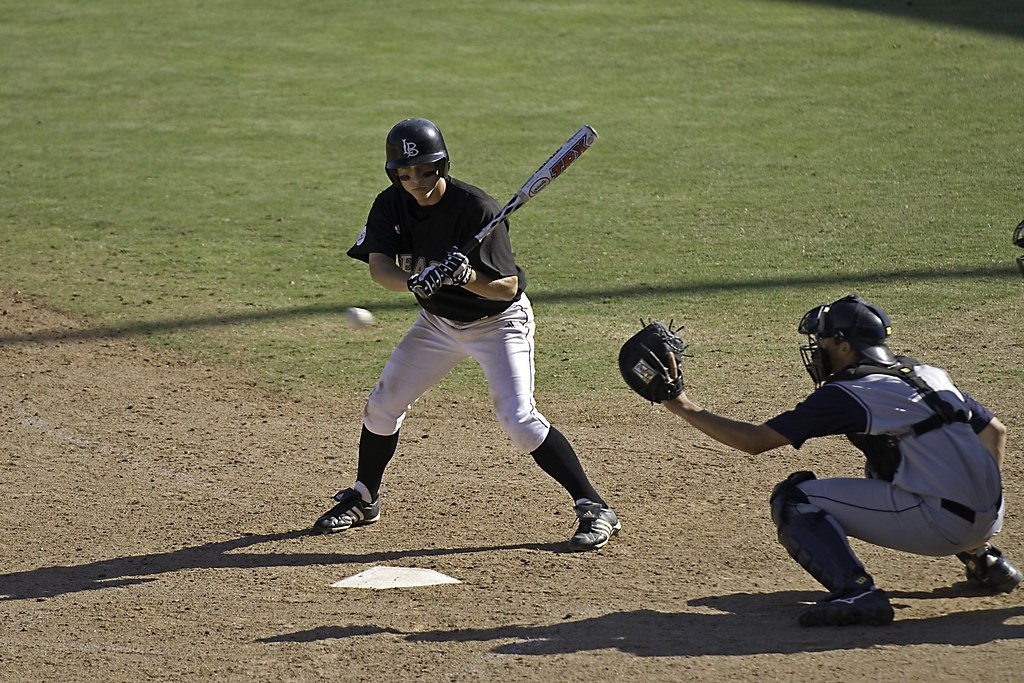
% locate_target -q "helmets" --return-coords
[806,294,892,343]
[384,118,450,190]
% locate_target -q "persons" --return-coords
[619,294,1022,628]
[313,118,621,551]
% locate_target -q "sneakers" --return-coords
[314,488,381,534]
[568,504,621,550]
[964,546,1023,593]
[798,589,894,627]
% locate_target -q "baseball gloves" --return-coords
[617,316,695,407]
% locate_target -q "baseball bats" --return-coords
[414,121,601,302]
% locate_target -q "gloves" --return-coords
[407,265,445,300]
[439,246,472,286]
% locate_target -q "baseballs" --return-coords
[346,306,373,329]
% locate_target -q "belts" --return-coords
[941,492,1003,524]
